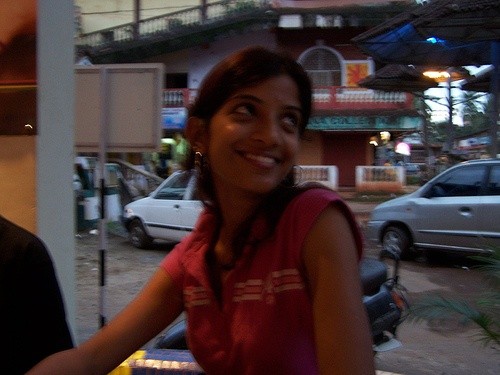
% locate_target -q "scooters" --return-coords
[152,243,412,358]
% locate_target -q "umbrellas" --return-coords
[358,64,438,94]
[351,0,500,64]
[461,65,500,91]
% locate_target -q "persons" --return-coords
[383,152,448,182]
[25,47,375,375]
[150,158,163,177]
[0,216,75,375]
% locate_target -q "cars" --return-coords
[369,158,500,266]
[119,169,204,249]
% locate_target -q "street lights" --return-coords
[422,71,452,158]
